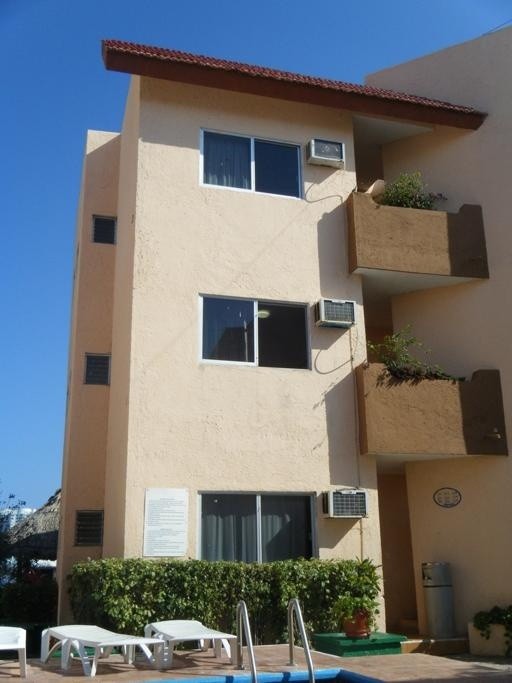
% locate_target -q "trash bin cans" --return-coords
[422,559,456,639]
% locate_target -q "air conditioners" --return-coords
[313,293,357,329]
[306,136,347,169]
[321,484,369,520]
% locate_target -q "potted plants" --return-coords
[330,591,380,639]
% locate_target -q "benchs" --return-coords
[0,618,237,677]
